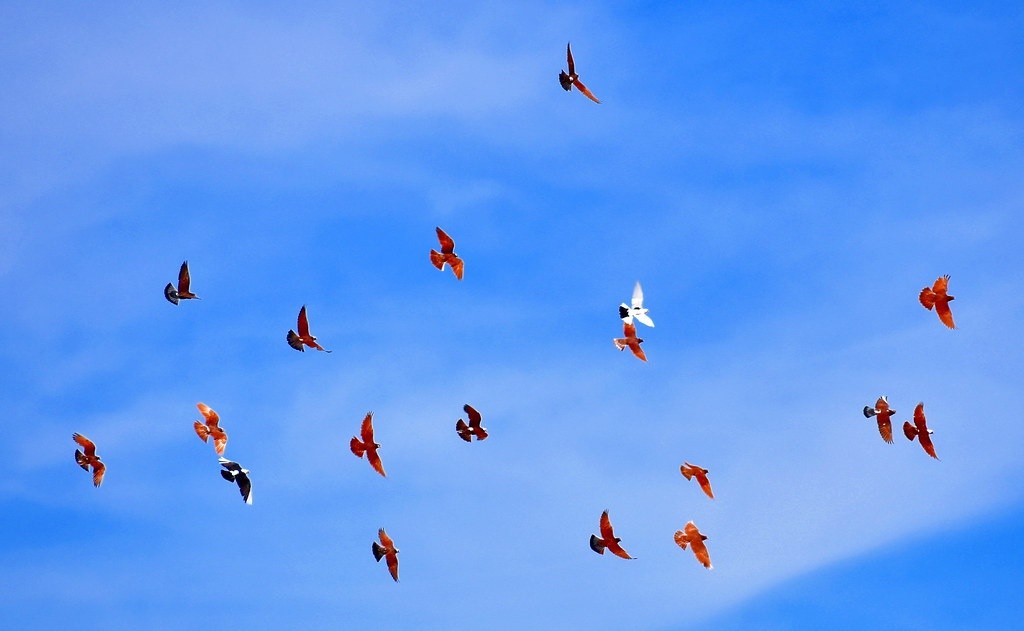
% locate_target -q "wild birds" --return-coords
[430,226,464,281]
[72,433,106,488]
[455,403,488,443]
[372,527,400,584]
[862,396,897,445]
[193,402,228,457]
[589,509,633,560]
[674,521,713,570]
[919,275,956,331]
[217,456,253,506]
[618,281,655,328]
[164,259,201,306]
[902,402,939,461]
[613,322,648,362]
[558,42,601,105]
[680,461,715,500]
[286,305,325,353]
[349,411,387,478]
